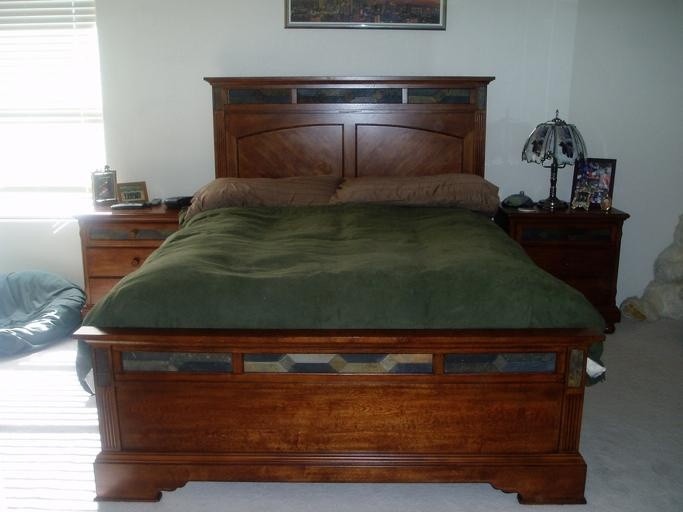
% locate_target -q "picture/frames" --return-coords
[569,158,617,211]
[92,170,117,205]
[117,181,149,204]
[283,0,448,31]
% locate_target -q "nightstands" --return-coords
[74,202,181,320]
[495,202,631,335]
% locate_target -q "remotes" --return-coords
[110,201,145,208]
[152,198,162,205]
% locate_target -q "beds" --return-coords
[70,74,606,506]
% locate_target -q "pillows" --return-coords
[333,173,499,214]
[185,175,339,221]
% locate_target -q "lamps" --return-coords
[522,108,589,211]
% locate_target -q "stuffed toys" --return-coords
[620,214,683,322]
